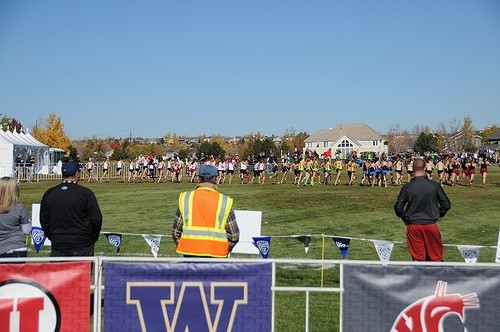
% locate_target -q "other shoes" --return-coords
[87,178,199,183]
[217,177,401,187]
[439,178,486,188]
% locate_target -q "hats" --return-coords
[198,165,218,177]
[62,163,83,177]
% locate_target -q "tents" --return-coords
[0,123,50,176]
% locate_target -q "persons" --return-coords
[480,156,491,187]
[406,150,479,187]
[61,152,82,181]
[172,165,238,257]
[128,147,358,186]
[26,155,35,181]
[114,158,123,176]
[39,162,102,262]
[100,158,110,182]
[86,157,96,183]
[361,151,406,187]
[0,176,31,264]
[16,153,23,182]
[479,147,500,164]
[394,159,452,261]
